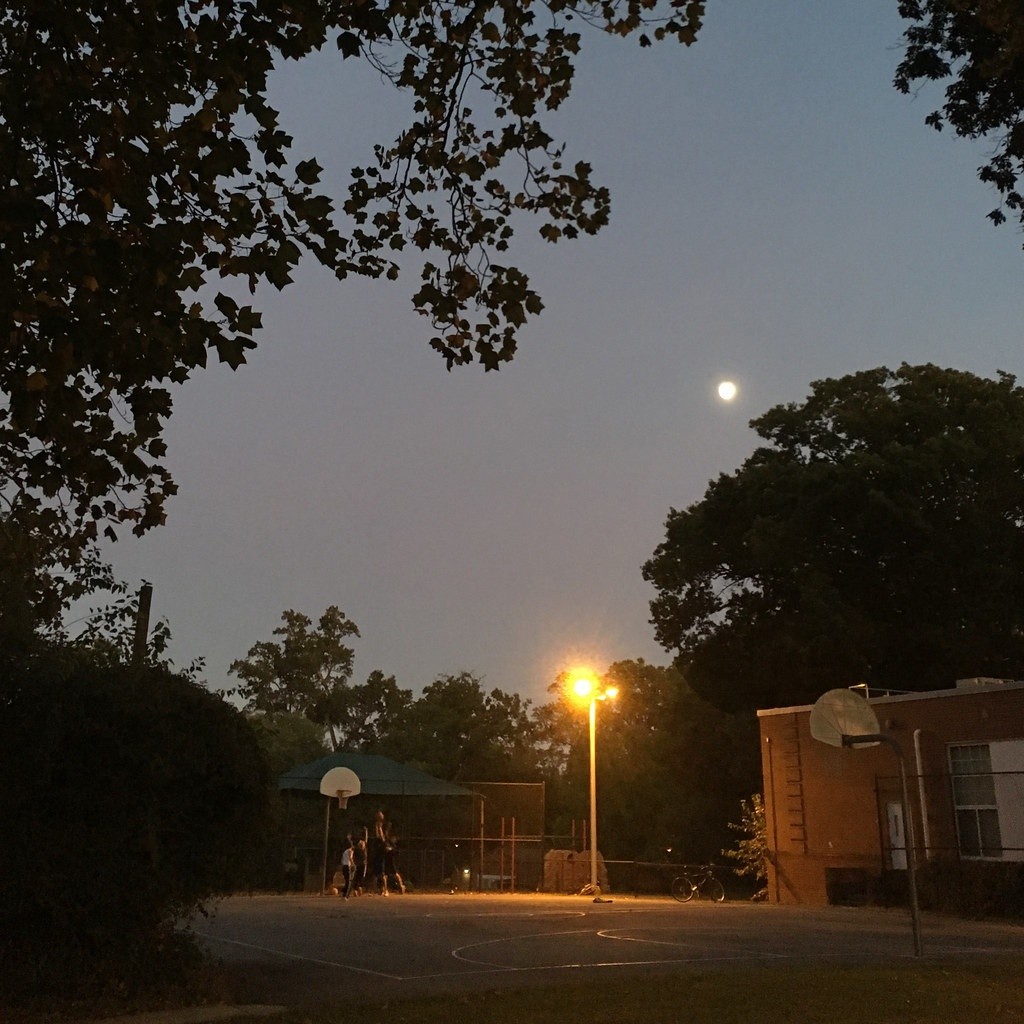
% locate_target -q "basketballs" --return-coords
[375,810,384,821]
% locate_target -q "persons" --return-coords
[342,820,406,901]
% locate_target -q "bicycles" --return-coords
[670,863,725,903]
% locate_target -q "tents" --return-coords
[268,753,488,895]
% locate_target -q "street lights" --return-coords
[572,677,619,896]
